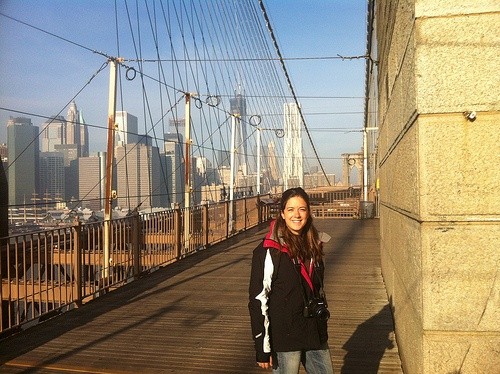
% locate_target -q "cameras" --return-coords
[304,297,330,323]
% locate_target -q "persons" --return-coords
[248,187,335,373]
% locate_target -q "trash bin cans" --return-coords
[359,201,375,220]
